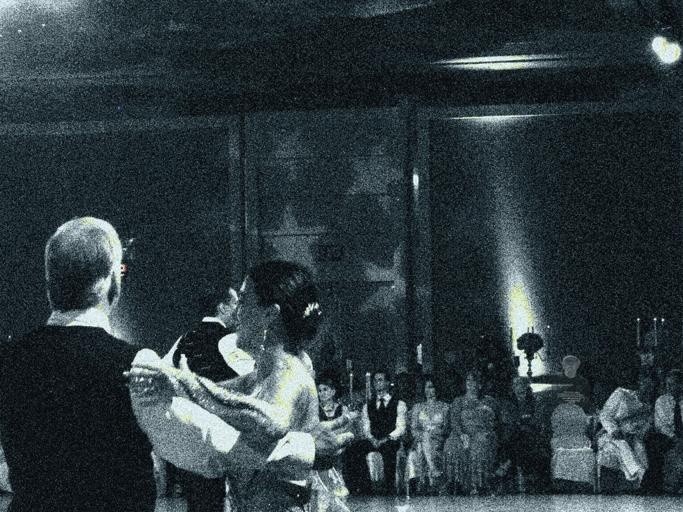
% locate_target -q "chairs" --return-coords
[365,403,603,494]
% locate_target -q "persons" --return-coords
[314,344,542,499]
[0,215,359,511]
[158,286,254,511]
[125,259,350,511]
[549,355,682,496]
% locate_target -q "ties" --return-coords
[379,398,385,409]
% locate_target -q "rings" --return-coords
[147,377,153,388]
